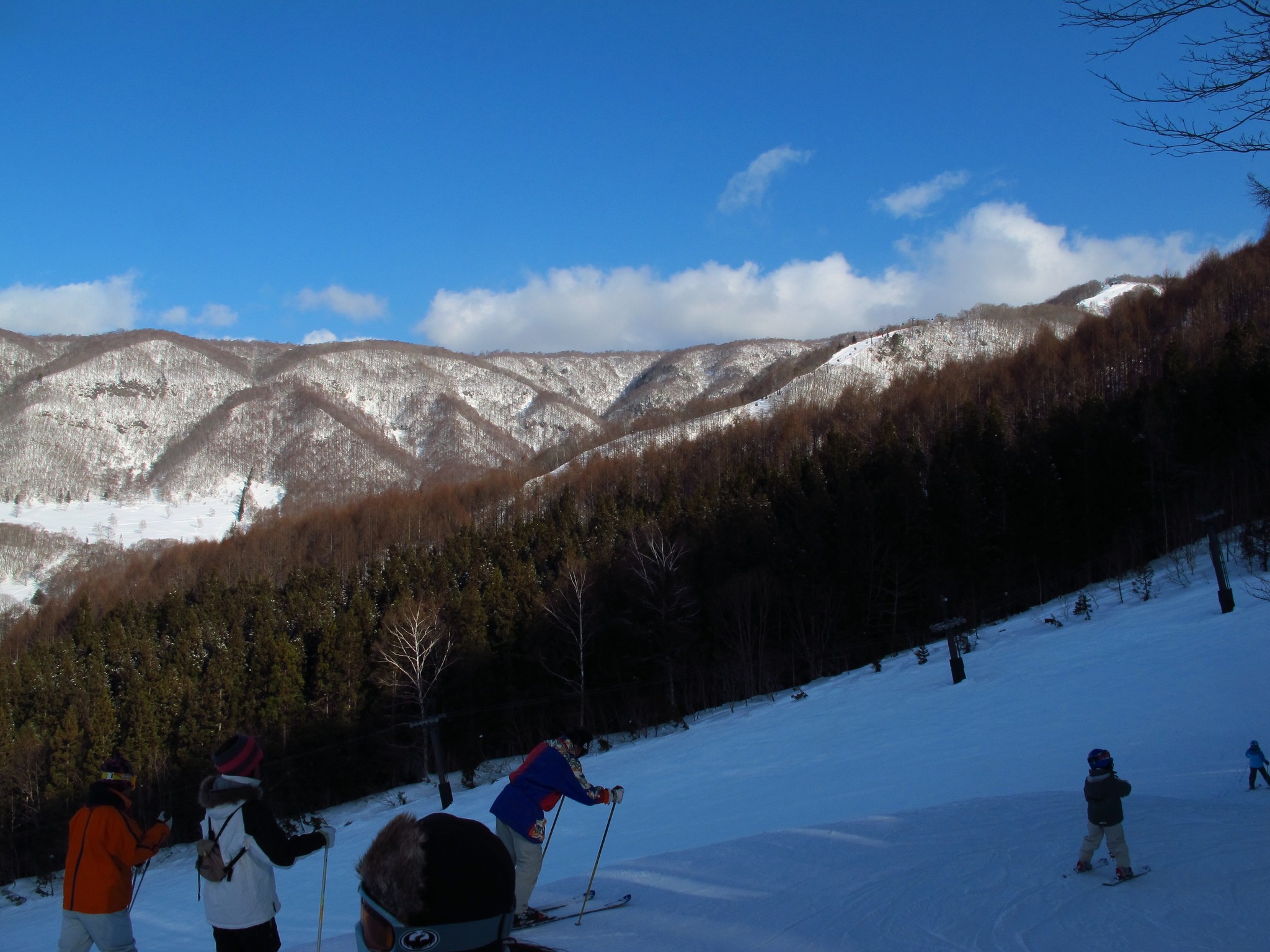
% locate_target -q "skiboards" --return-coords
[509,890,633,928]
[1064,856,1154,885]
[1243,783,1270,794]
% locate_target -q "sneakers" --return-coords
[512,906,547,929]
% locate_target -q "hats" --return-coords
[213,734,263,777]
[565,727,593,759]
[98,756,134,790]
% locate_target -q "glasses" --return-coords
[355,882,404,952]
[131,775,137,790]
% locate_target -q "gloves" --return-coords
[606,786,624,805]
[316,825,337,848]
[157,808,172,831]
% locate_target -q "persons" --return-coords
[198,733,335,952]
[489,726,624,926]
[56,747,173,952]
[1245,740,1270,789]
[355,813,561,952]
[447,723,483,789]
[1075,748,1134,879]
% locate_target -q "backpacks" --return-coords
[195,806,247,882]
